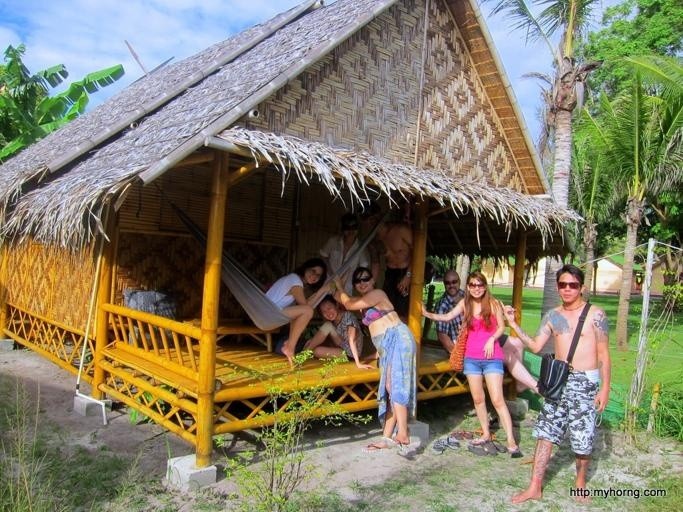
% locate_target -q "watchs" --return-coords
[405,271,413,277]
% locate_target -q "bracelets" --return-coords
[337,288,343,290]
[491,335,496,339]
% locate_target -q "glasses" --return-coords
[351,277,373,284]
[444,280,457,286]
[467,282,486,290]
[556,281,580,290]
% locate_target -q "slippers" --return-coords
[431,427,506,458]
[507,442,519,455]
[364,440,411,454]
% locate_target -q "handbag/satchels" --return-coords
[448,303,469,375]
[537,301,593,402]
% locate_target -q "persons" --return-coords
[302,295,375,369]
[503,264,612,504]
[435,270,539,395]
[377,222,414,326]
[320,211,369,297]
[332,266,417,451]
[264,256,328,368]
[415,271,520,454]
